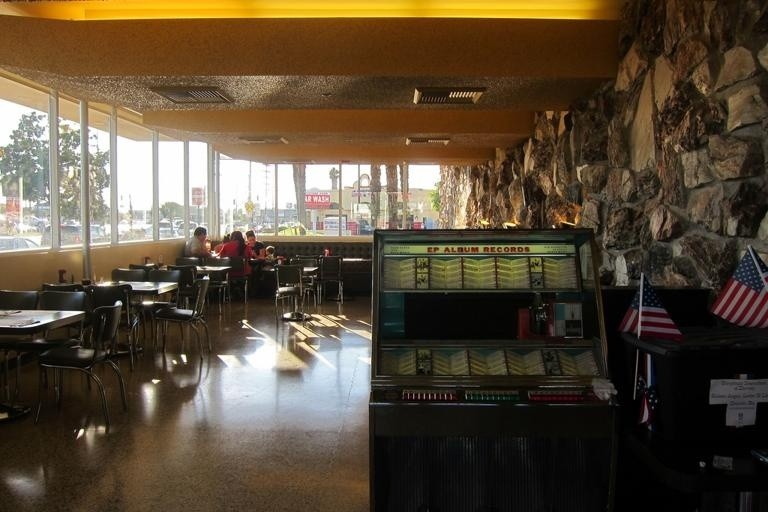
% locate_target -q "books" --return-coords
[519,300,583,340]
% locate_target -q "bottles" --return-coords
[144,256,150,263]
[323,246,332,256]
[276,255,284,265]
[58,268,67,284]
[99,276,104,284]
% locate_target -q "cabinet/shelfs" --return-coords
[367,227,616,512]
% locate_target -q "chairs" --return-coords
[151,274,212,361]
[41,267,184,373]
[33,300,128,435]
[127,241,343,328]
[2,289,87,423]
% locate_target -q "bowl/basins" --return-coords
[81,278,91,285]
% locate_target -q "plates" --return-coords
[144,264,154,266]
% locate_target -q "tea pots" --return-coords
[156,255,166,268]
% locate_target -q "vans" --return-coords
[41,224,106,248]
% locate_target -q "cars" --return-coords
[356,219,373,236]
[0,235,39,251]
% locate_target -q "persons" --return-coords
[183,226,276,291]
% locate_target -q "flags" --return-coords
[619,272,681,342]
[708,246,767,331]
[635,374,660,433]
